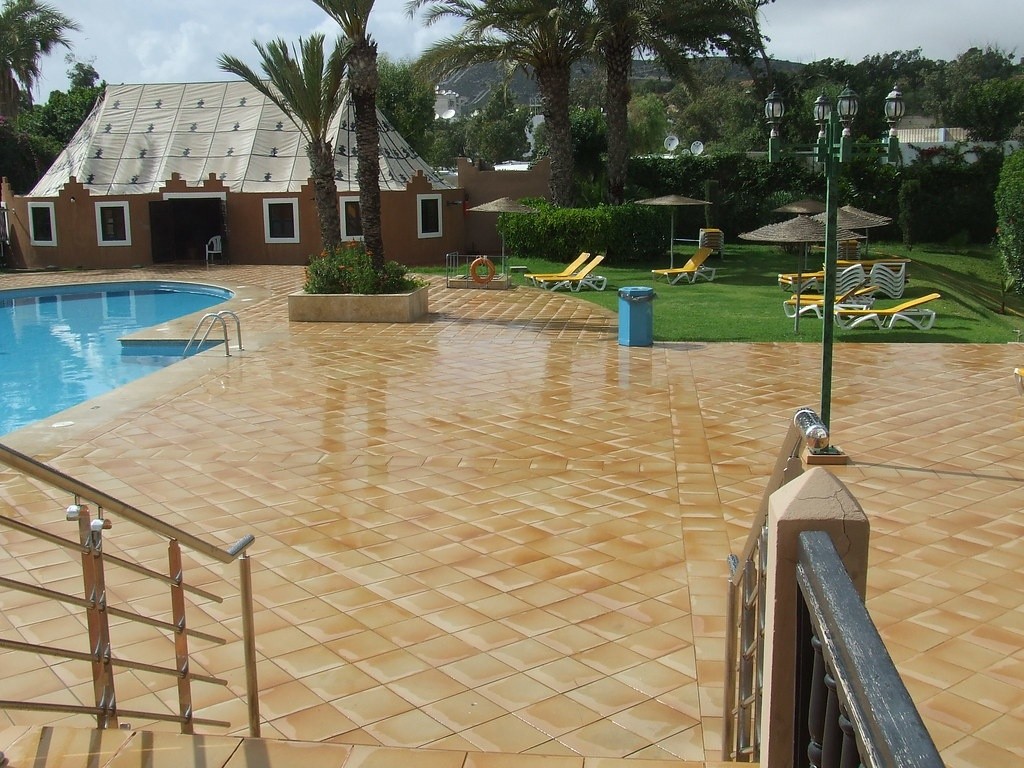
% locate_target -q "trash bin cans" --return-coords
[617,287,654,347]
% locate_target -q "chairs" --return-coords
[699,228,723,259]
[536,255,607,292]
[205,235,223,263]
[652,246,715,286]
[524,252,591,287]
[780,240,941,331]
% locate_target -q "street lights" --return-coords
[764,79,907,466]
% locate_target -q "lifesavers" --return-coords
[470,257,496,284]
